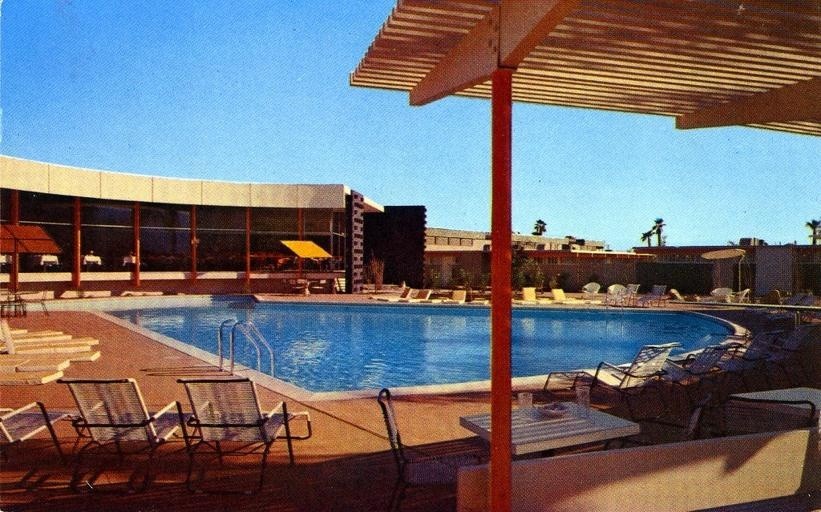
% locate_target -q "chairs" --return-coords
[372,288,487,304]
[637,286,665,308]
[734,290,750,308]
[377,389,480,512]
[0,400,87,493]
[644,392,713,442]
[26,284,52,319]
[622,284,639,309]
[58,378,194,492]
[605,284,626,306]
[581,282,600,303]
[592,323,821,410]
[176,377,313,498]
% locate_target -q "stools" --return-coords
[728,388,818,429]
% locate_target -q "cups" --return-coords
[573,384,592,419]
[516,391,535,426]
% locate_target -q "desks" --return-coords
[459,401,640,456]
[0,288,29,320]
[710,288,731,305]
